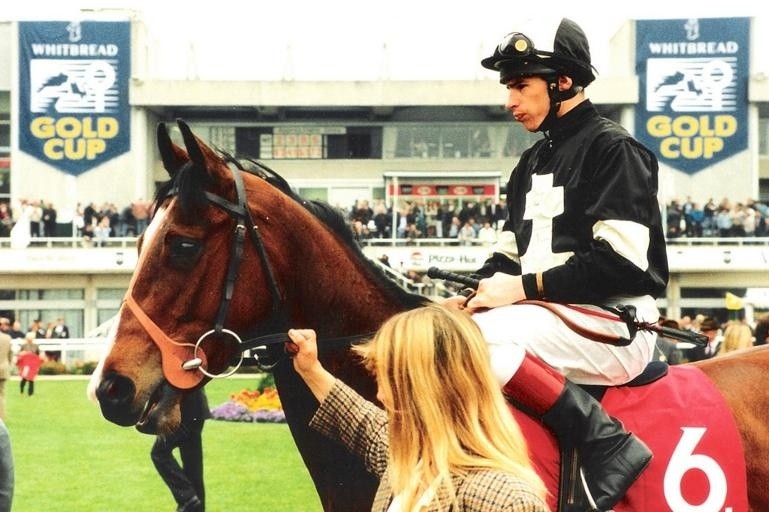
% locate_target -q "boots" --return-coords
[539,375,654,511]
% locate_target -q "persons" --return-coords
[655,310,769,362]
[441,16,672,512]
[277,304,552,512]
[666,193,769,244]
[0,192,154,248]
[149,386,214,511]
[344,194,509,245]
[0,316,69,395]
[0,416,16,511]
[381,254,455,297]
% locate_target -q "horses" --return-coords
[87,116,769,512]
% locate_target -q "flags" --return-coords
[634,12,753,175]
[13,10,134,177]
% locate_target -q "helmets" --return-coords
[481,17,595,82]
